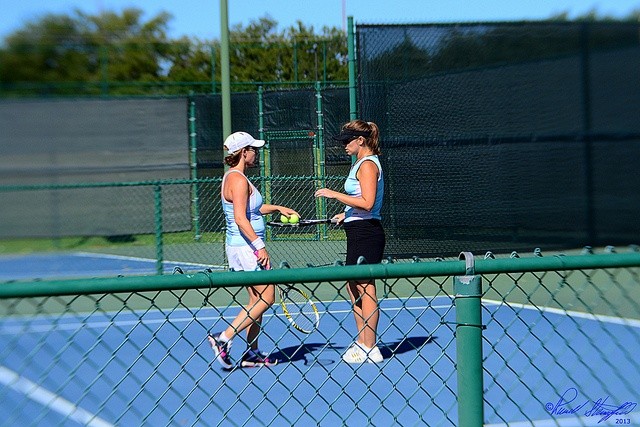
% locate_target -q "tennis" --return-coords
[280,214,289,224]
[289,215,299,224]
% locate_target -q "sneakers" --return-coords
[342,340,384,363]
[208,332,232,369]
[240,348,279,368]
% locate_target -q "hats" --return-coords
[332,130,372,141]
[223,131,265,155]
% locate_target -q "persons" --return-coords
[313,120,386,364]
[208,132,300,369]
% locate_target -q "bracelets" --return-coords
[251,238,265,250]
